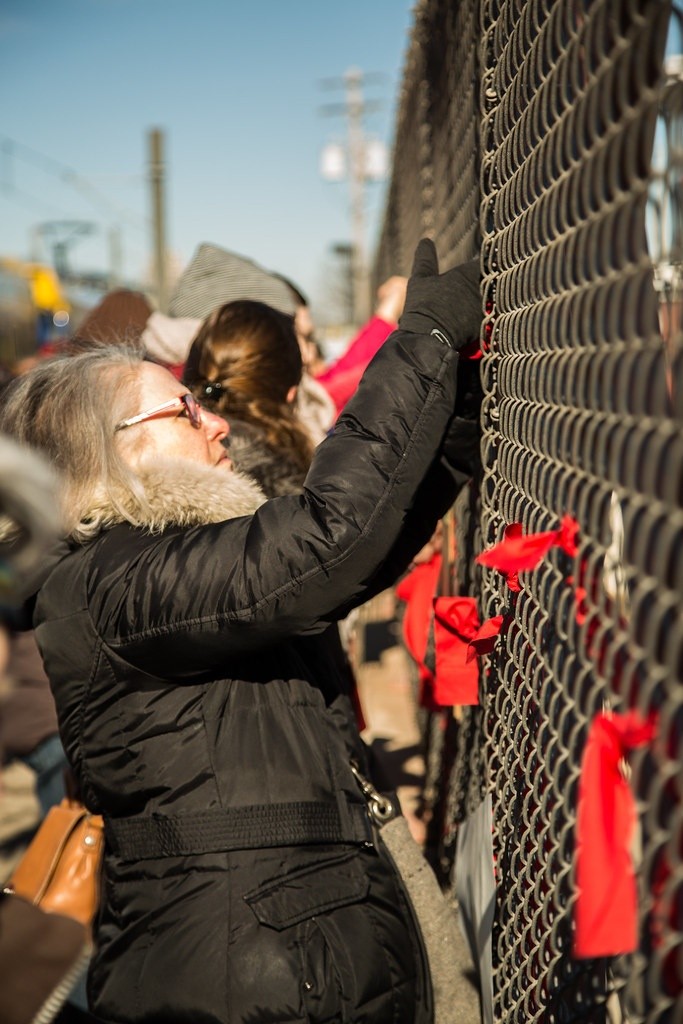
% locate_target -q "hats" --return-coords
[170,242,297,321]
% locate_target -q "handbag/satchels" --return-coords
[0,800,103,928]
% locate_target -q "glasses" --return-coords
[115,393,203,432]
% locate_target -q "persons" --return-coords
[0,237,484,1023]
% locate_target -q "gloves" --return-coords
[397,238,483,349]
[451,352,496,420]
